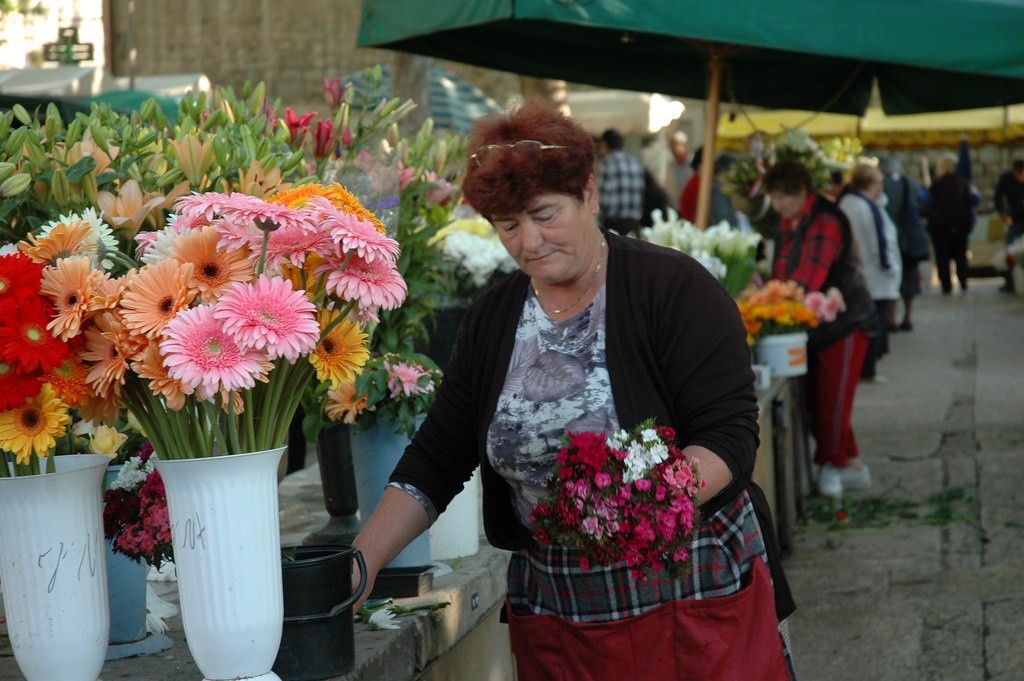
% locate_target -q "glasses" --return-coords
[470,140,571,168]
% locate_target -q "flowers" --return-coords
[725,128,879,238]
[0,65,522,632]
[608,208,845,348]
[527,417,707,582]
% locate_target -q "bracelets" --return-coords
[385,482,439,529]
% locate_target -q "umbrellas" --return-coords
[320,58,503,133]
[714,76,1024,153]
[357,0,1024,234]
[22,88,180,128]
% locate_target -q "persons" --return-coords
[594,129,1024,382]
[759,158,873,498]
[352,99,795,681]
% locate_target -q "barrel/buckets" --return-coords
[271,544,367,681]
[757,332,808,379]
[351,415,479,563]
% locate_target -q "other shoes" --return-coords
[839,465,871,491]
[818,465,842,499]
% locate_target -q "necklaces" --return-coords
[532,234,604,313]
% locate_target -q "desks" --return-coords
[1,461,521,680]
[751,376,814,557]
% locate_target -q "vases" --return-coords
[103,463,172,662]
[348,412,431,567]
[431,464,480,559]
[0,455,112,680]
[152,446,288,681]
